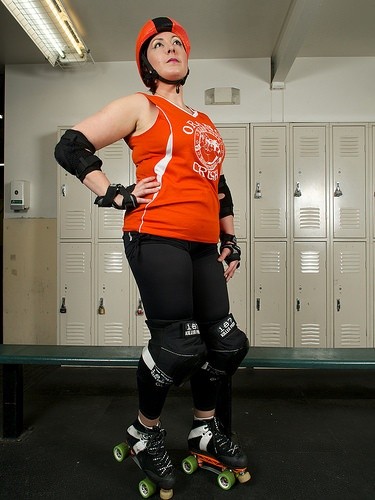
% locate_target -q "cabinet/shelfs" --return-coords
[55,122,375,349]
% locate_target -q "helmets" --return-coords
[136,16,191,88]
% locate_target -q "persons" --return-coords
[53,15,250,500]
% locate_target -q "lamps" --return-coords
[0,0,94,70]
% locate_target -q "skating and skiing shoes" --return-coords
[181,413,251,491]
[113,416,179,500]
